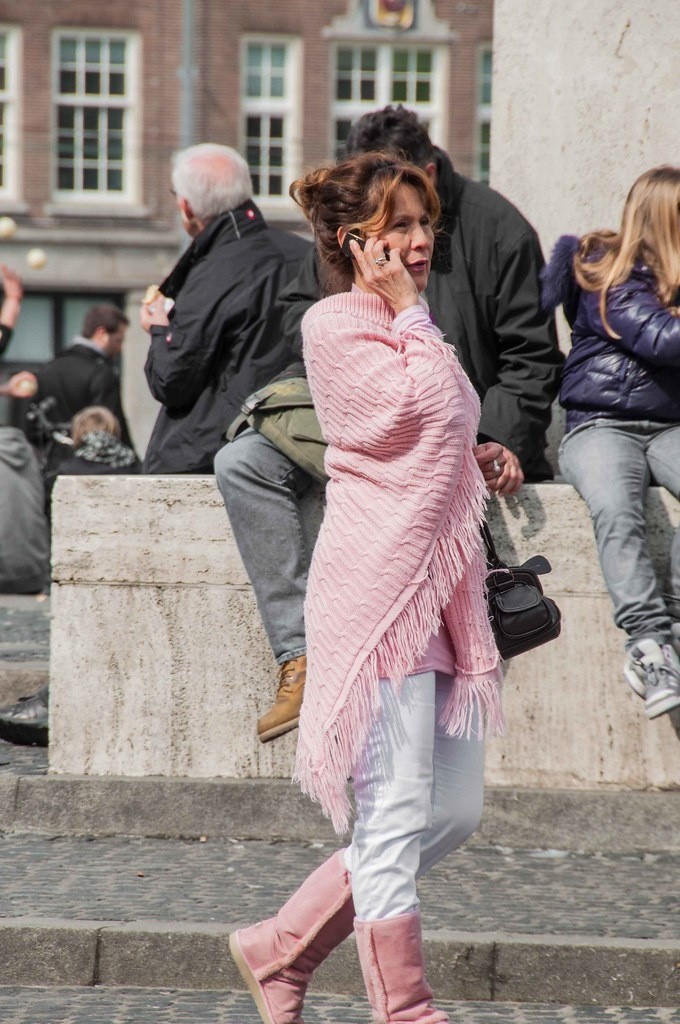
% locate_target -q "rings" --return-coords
[375,257,388,266]
[492,459,500,472]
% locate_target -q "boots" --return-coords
[353,909,452,1024]
[228,848,359,1023]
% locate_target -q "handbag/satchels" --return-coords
[478,520,563,660]
[224,378,329,484]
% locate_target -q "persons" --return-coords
[214,103,568,746]
[0,257,50,594]
[45,405,143,522]
[143,142,336,476]
[229,150,540,1023]
[26,306,145,463]
[540,164,680,720]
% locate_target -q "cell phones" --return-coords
[341,231,391,261]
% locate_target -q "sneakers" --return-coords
[257,655,307,744]
[630,637,680,719]
[623,623,680,699]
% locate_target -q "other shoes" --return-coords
[0,685,49,744]
